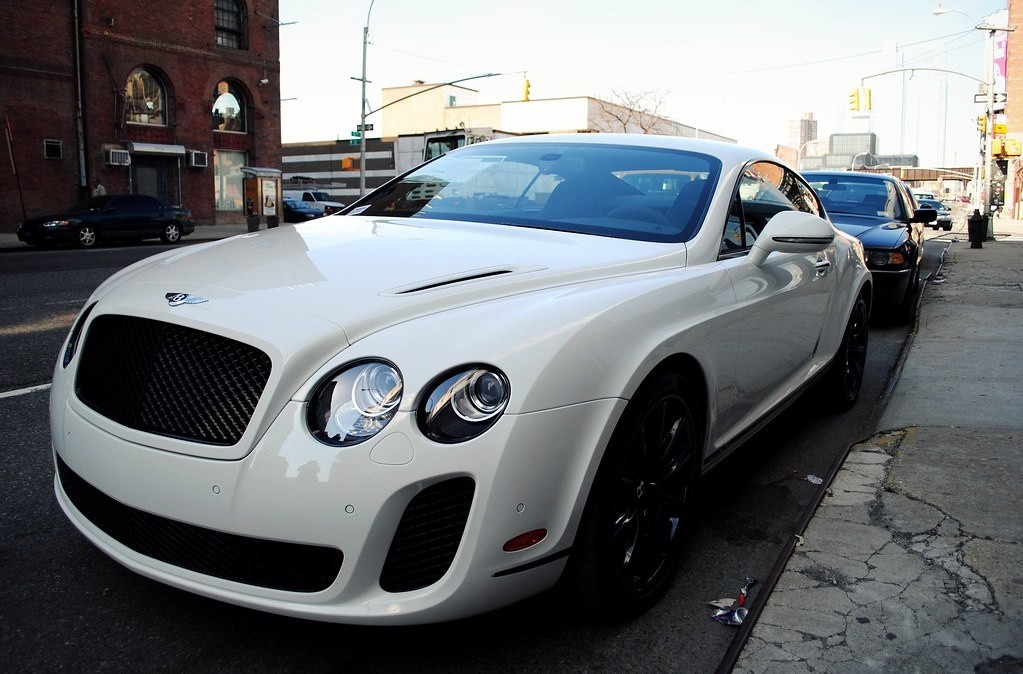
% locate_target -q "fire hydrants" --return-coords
[969,209,983,249]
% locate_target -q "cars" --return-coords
[916,199,953,231]
[796,170,937,327]
[48,133,874,627]
[16,194,194,249]
[283,198,323,224]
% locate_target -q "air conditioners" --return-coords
[188,150,208,167]
[105,148,130,167]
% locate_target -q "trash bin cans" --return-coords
[968,218,989,242]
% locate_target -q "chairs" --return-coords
[540,179,622,224]
[863,194,888,212]
[663,178,747,251]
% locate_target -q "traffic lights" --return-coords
[524,80,530,101]
[977,115,986,134]
[849,89,859,111]
[864,88,872,109]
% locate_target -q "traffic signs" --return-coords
[974,92,1008,104]
[357,124,373,131]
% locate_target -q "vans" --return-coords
[282,189,346,216]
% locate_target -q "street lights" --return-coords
[851,152,868,171]
[797,140,818,170]
[934,9,996,241]
